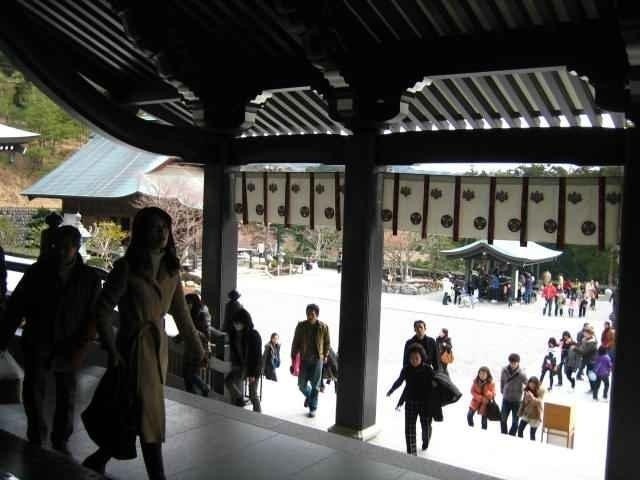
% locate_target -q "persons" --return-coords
[386,343,438,456]
[262,333,281,382]
[435,328,452,377]
[0,224,102,457]
[440,262,618,319]
[78,206,209,479]
[516,374,544,439]
[290,303,330,418]
[402,320,441,380]
[326,346,336,385]
[539,320,616,402]
[466,366,496,429]
[499,353,530,436]
[223,308,263,414]
[169,294,213,398]
[254,238,343,274]
[318,361,326,392]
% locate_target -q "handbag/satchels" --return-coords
[81,361,136,460]
[486,400,500,421]
[441,352,453,363]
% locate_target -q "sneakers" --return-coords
[53,445,72,455]
[547,376,584,390]
[235,397,246,407]
[305,396,316,417]
[589,389,608,402]
